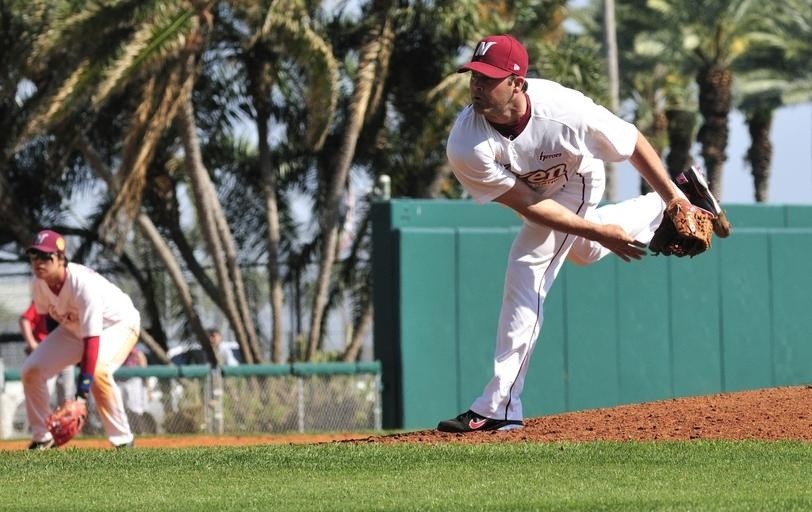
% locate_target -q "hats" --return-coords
[26,230,66,255]
[457,34,528,79]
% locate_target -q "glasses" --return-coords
[28,250,51,261]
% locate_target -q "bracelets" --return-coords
[78,374,93,393]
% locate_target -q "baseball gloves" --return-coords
[45,403,88,446]
[649,199,714,258]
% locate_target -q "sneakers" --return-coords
[29,437,56,448]
[674,166,730,238]
[438,410,525,432]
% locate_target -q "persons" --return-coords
[435,35,733,432]
[21,230,142,448]
[21,294,79,415]
[111,328,240,434]
